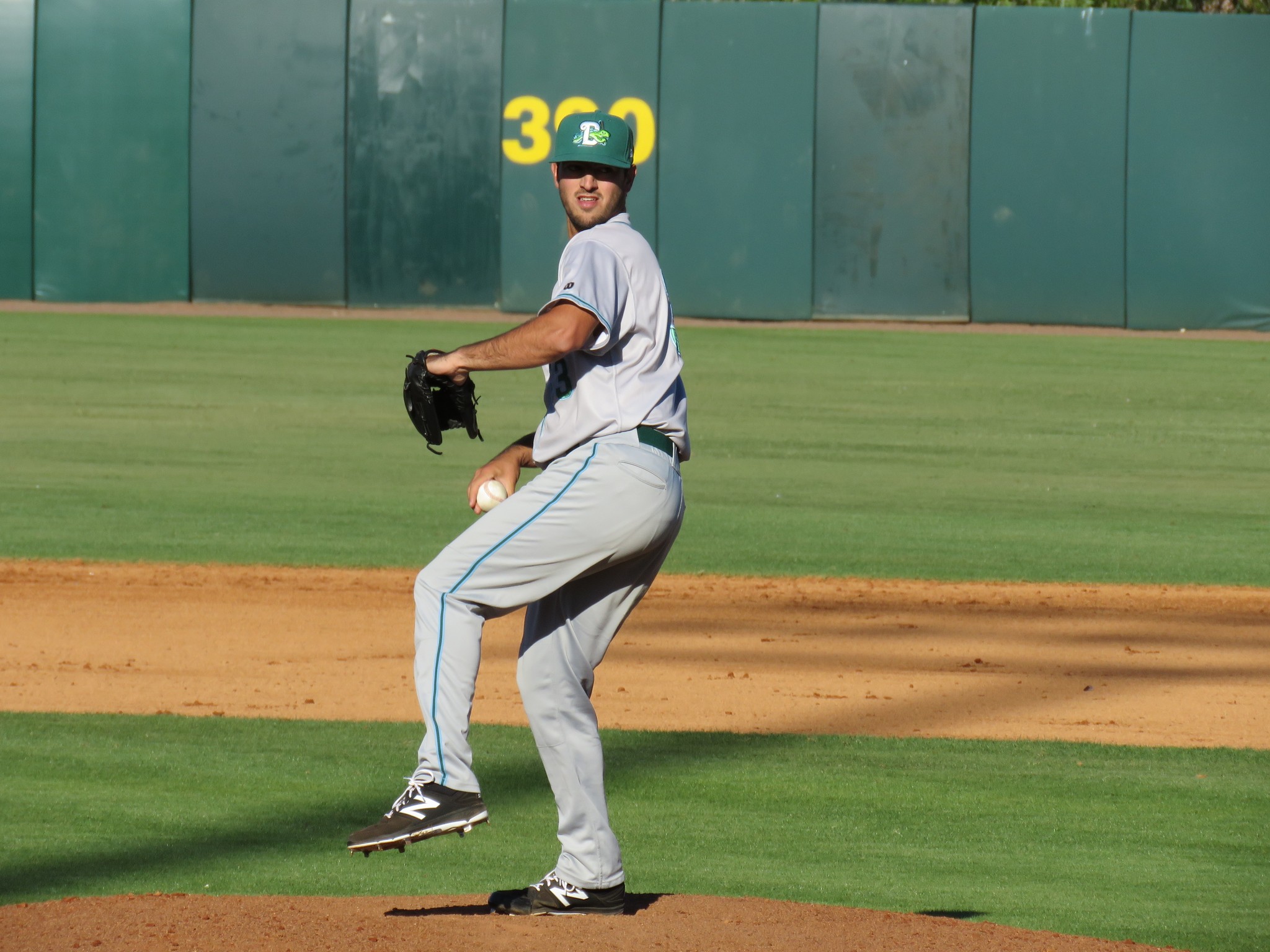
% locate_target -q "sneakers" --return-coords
[489,867,627,915]
[346,770,490,853]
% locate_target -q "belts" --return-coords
[637,426,683,462]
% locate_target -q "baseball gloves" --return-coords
[403,347,484,447]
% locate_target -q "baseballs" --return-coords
[475,477,507,511]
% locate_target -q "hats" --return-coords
[549,110,637,169]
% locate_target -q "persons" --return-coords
[347,110,689,916]
